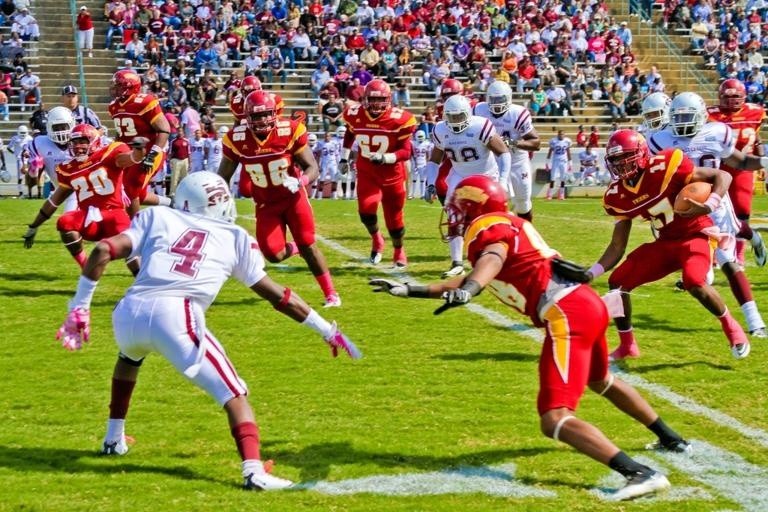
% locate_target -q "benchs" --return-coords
[1,0,767,150]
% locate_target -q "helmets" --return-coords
[451,175,508,218]
[18,106,101,153]
[719,79,747,98]
[177,171,232,219]
[643,92,707,125]
[219,126,229,138]
[336,126,346,137]
[487,81,512,105]
[415,130,426,141]
[110,70,141,95]
[605,129,650,169]
[364,79,391,103]
[425,185,436,202]
[242,76,276,128]
[308,134,316,145]
[442,79,471,120]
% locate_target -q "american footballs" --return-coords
[673,181,714,213]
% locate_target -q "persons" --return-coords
[56,170,362,492]
[366,174,695,502]
[0,0,767,361]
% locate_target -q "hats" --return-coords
[63,85,78,95]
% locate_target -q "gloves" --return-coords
[23,226,36,248]
[369,279,409,296]
[129,137,160,174]
[339,163,350,175]
[121,184,131,207]
[283,172,300,194]
[324,321,361,359]
[370,153,384,164]
[56,307,89,350]
[434,288,472,314]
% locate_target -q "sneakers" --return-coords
[324,293,341,308]
[442,266,465,279]
[753,231,766,267]
[103,441,128,455]
[371,239,406,268]
[729,320,765,357]
[648,440,694,457]
[244,472,295,490]
[613,471,671,502]
[608,346,639,360]
